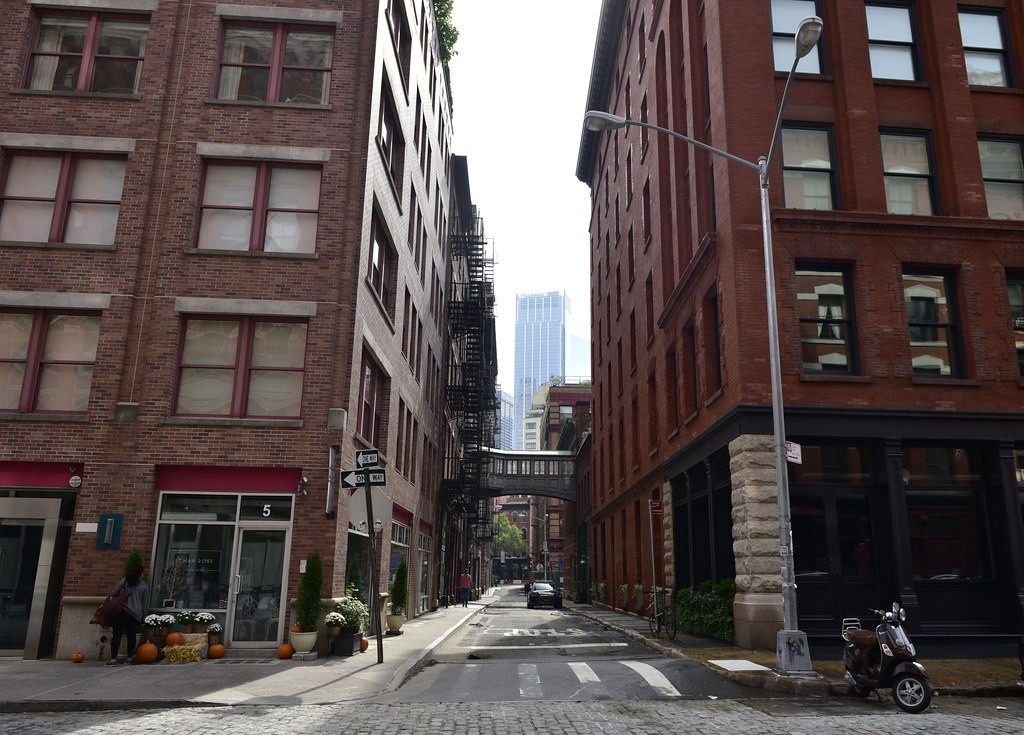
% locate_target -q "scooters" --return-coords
[842,601,932,714]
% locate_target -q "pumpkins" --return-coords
[72,652,84,663]
[208,643,225,658]
[136,639,157,663]
[166,632,181,646]
[277,643,295,659]
[361,637,368,652]
[291,624,301,632]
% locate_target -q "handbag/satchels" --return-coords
[89,597,113,627]
[100,577,132,620]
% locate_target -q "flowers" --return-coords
[324,612,347,627]
[206,624,223,633]
[177,611,215,624]
[144,614,176,627]
[338,584,369,626]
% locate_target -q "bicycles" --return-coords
[642,590,677,641]
[583,16,825,677]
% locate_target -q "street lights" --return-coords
[516,513,549,581]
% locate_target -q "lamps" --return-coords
[580,554,591,566]
[296,477,308,497]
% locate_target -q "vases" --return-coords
[159,627,170,636]
[183,623,192,634]
[341,626,361,634]
[195,624,207,633]
[328,627,341,635]
[209,635,219,645]
[146,628,156,637]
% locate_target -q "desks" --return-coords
[254,608,273,641]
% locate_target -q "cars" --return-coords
[527,582,562,610]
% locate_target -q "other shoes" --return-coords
[104,661,117,667]
[124,661,132,665]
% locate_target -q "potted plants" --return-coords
[290,551,324,654]
[117,545,145,657]
[385,557,409,633]
[618,583,663,614]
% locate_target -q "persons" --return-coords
[104,566,150,666]
[460,568,472,608]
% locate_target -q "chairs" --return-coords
[235,597,259,641]
[264,596,281,641]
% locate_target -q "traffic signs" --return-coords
[356,449,380,469]
[340,468,386,489]
